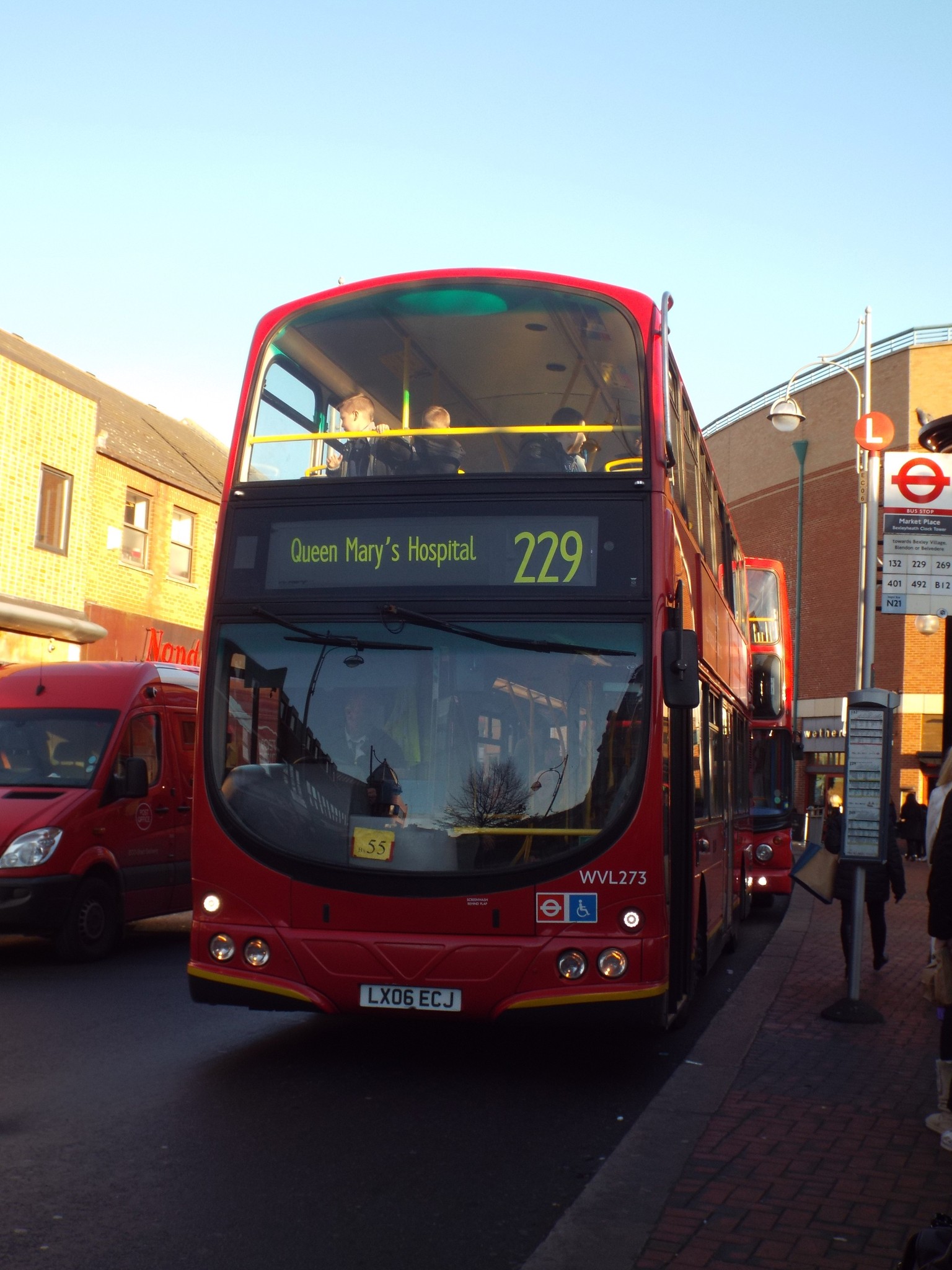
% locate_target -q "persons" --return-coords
[924,745,952,1152]
[597,431,643,472]
[325,393,414,478]
[888,793,926,862]
[824,813,906,981]
[410,405,466,475]
[510,739,530,772]
[511,406,590,472]
[542,738,564,770]
[310,689,409,829]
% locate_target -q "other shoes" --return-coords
[940,1131,952,1151]
[925,1113,952,1134]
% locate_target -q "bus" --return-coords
[741,546,799,910]
[179,270,754,1036]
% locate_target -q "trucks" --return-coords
[1,660,208,957]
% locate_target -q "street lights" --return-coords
[774,304,892,1025]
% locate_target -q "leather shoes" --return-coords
[873,953,889,971]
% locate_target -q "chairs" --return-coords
[51,740,85,779]
